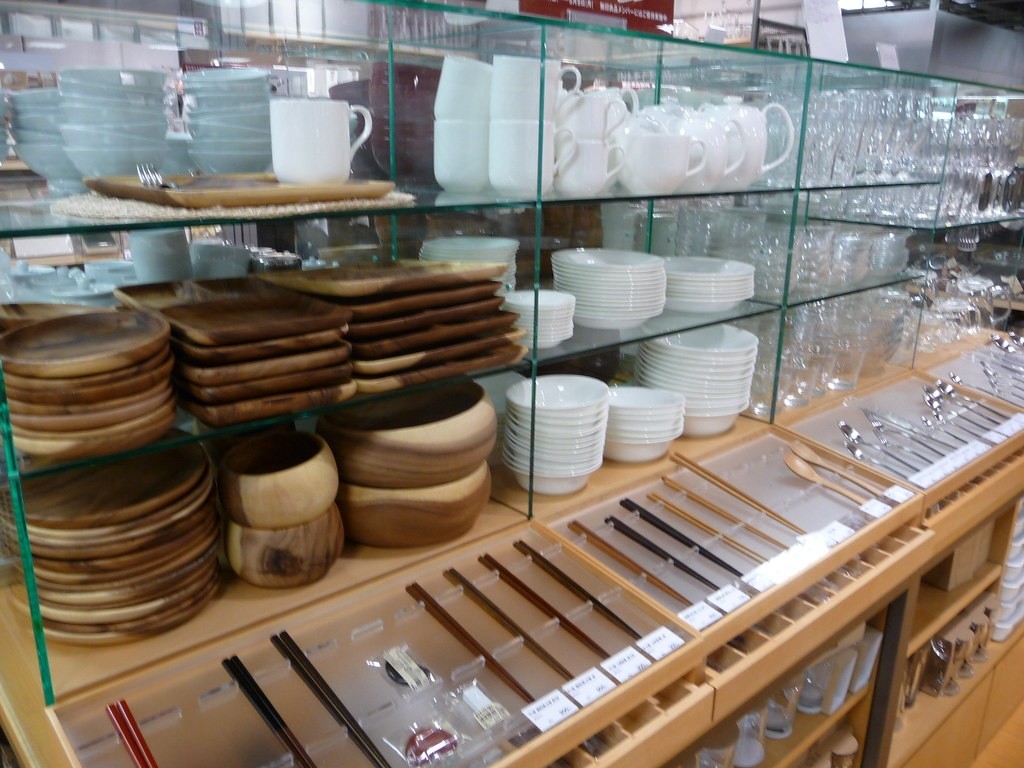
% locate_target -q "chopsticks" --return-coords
[101,450,808,768]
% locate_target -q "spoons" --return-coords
[783,440,882,506]
[832,331,1024,479]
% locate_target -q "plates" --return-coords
[0,226,382,312]
[421,233,764,496]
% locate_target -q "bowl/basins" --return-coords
[0,63,269,194]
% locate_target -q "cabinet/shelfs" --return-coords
[0,0,1024,709]
[663,491,1024,768]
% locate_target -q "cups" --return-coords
[430,53,794,196]
[740,85,1024,415]
[266,97,372,187]
